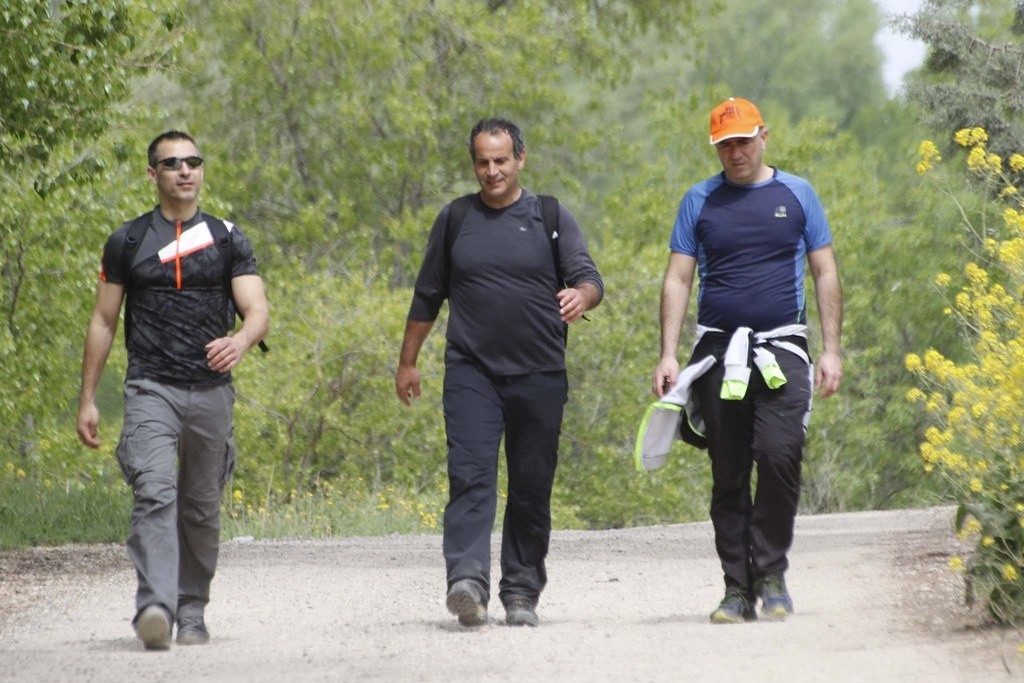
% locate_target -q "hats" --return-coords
[710,97,764,146]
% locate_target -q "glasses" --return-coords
[154,156,203,170]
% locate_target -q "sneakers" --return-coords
[446,582,487,626]
[504,599,538,625]
[135,604,173,650]
[709,594,757,622]
[176,616,209,644]
[753,572,794,615]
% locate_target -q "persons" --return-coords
[394,119,603,627]
[650,97,841,623]
[74,130,268,651]
